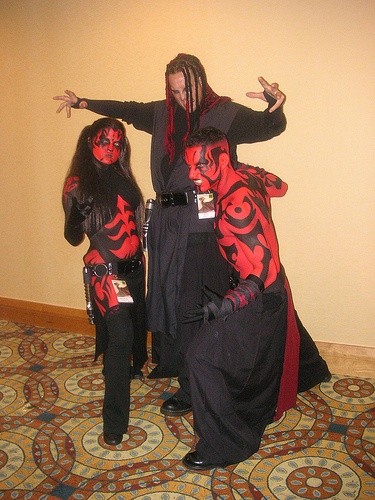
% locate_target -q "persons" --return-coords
[53,53,287,414]
[184,128,331,470]
[62,118,147,446]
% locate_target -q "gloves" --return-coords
[180,284,235,324]
[67,197,95,229]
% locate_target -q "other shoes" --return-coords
[102,366,144,379]
[147,367,171,379]
[103,424,123,446]
[133,356,148,370]
[161,387,194,416]
[182,450,219,471]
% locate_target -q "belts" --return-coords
[84,240,144,279]
[154,188,199,209]
